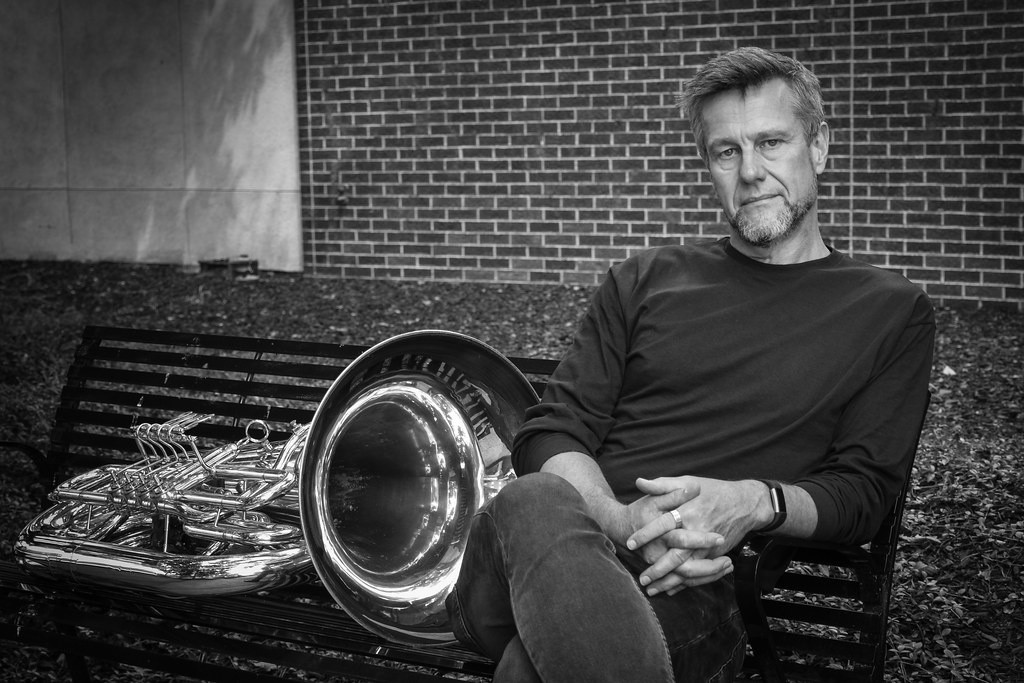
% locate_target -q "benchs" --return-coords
[0,326,933,683]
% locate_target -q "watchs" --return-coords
[752,478,787,537]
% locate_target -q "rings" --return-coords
[670,509,683,529]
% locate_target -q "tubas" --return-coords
[11,329,543,651]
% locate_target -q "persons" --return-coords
[444,47,936,683]
